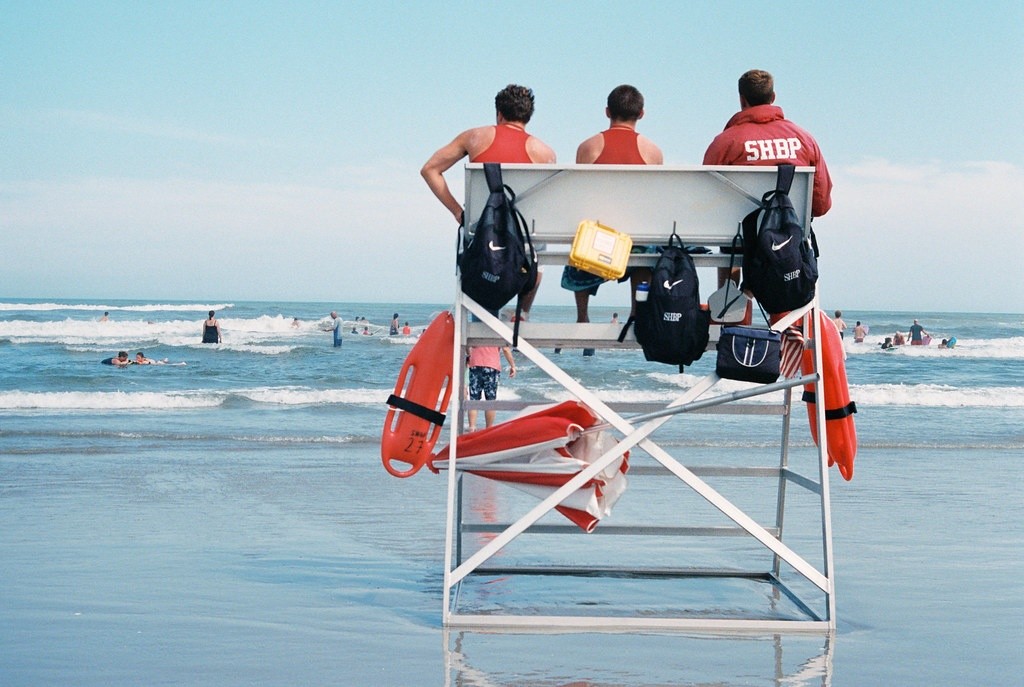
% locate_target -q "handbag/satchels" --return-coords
[715,324,781,383]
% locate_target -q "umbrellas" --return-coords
[427,373,820,533]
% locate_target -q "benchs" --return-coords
[443,162,837,632]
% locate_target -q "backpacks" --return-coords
[456,162,538,310]
[635,233,709,365]
[740,165,819,313]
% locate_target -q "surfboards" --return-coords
[946,336,957,348]
[922,336,932,345]
[854,325,868,339]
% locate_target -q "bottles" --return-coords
[635,281,650,302]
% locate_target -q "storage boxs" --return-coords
[569,219,633,280]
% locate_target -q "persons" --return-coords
[852,321,866,343]
[403,322,410,334]
[561,85,663,323]
[611,313,618,324]
[356,317,366,322]
[420,84,557,321]
[136,352,186,365]
[351,327,369,335]
[893,331,905,345]
[292,318,298,328]
[390,313,400,335]
[938,339,947,349]
[202,310,222,343]
[908,319,928,345]
[881,337,892,349]
[102,312,109,321]
[326,312,343,347]
[466,346,515,432]
[102,351,129,366]
[703,70,832,302]
[833,310,847,340]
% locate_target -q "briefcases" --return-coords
[569,221,633,280]
